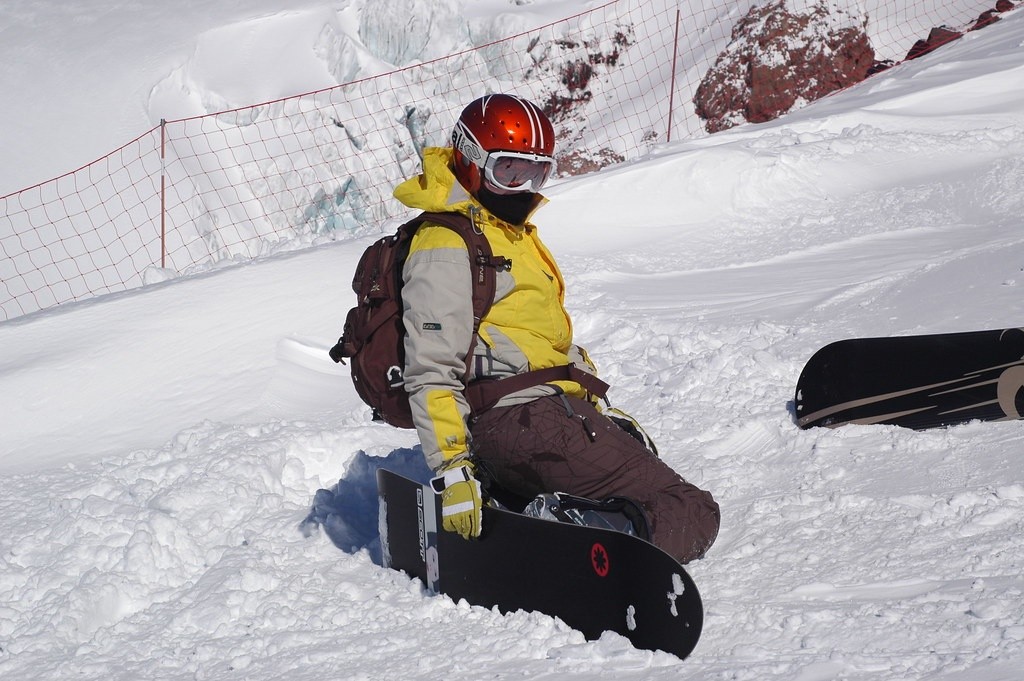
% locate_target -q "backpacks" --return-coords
[328,209,610,430]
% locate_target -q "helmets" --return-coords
[452,94,556,195]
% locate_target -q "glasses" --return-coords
[451,123,555,194]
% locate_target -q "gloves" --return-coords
[430,455,482,540]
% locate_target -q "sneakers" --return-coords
[525,493,620,532]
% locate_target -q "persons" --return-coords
[391,93,722,566]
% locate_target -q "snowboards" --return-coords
[373,468,704,663]
[793,326,1024,431]
